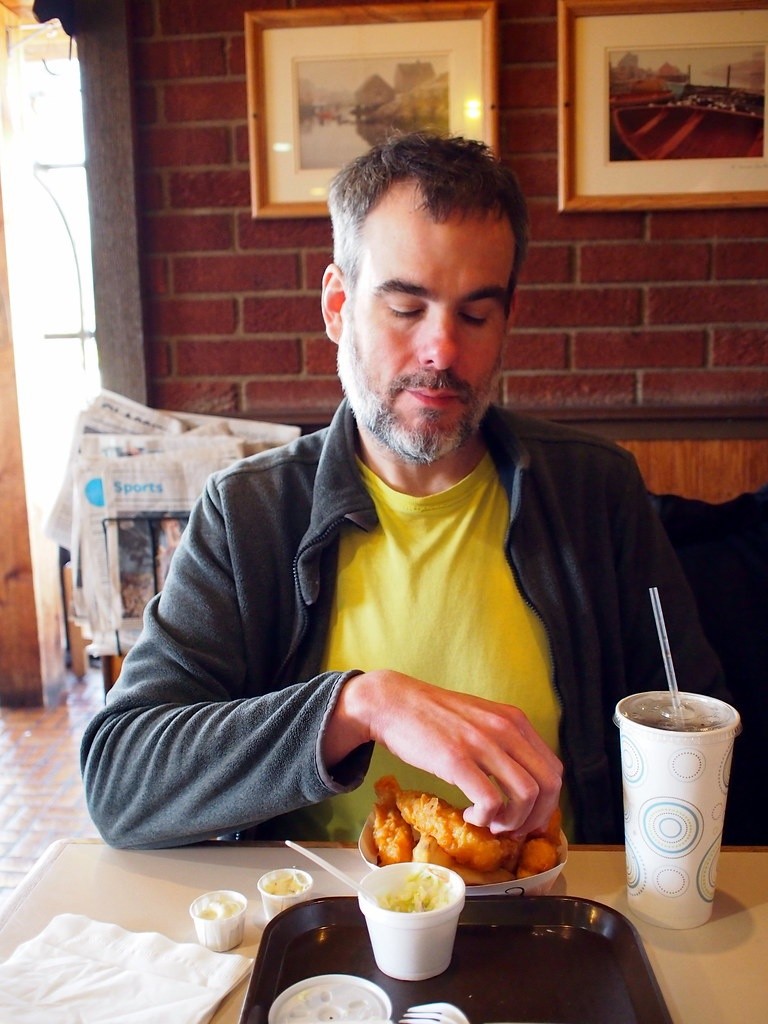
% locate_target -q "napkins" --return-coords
[1,911,254,1024]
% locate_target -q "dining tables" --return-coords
[1,837,768,1024]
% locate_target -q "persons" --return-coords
[81,129,768,850]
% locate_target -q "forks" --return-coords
[398,1003,469,1024]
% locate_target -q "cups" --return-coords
[359,862,467,982]
[190,890,248,952]
[259,868,314,920]
[612,691,740,931]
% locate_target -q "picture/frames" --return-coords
[241,0,500,222]
[556,0,768,214]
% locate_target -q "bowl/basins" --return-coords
[357,807,570,897]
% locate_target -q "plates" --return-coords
[267,974,391,1022]
